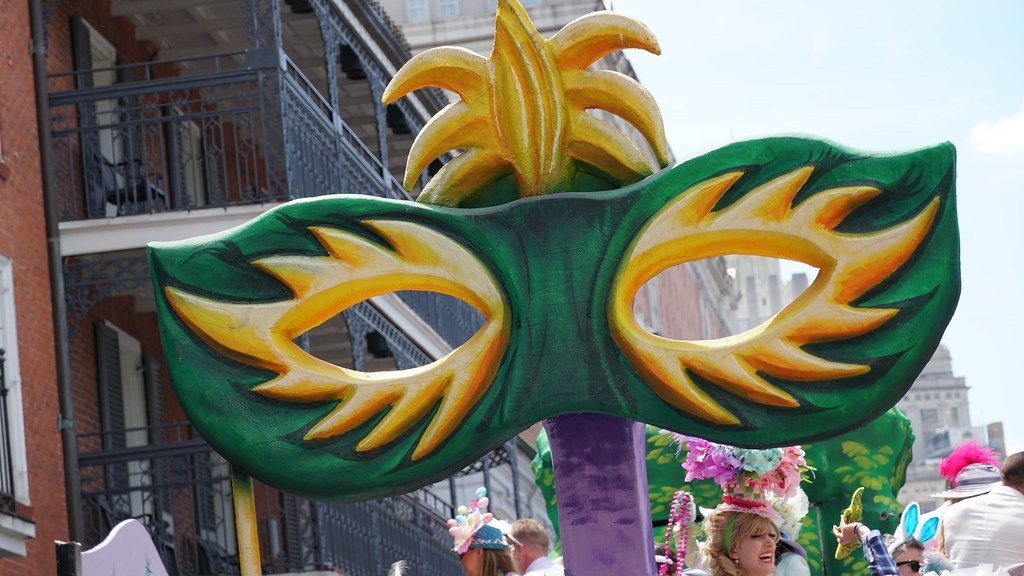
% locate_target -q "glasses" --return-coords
[897,560,921,572]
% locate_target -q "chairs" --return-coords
[101,154,170,216]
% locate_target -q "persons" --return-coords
[505,519,565,576]
[894,463,1003,553]
[833,513,926,576]
[653,543,712,576]
[448,487,518,576]
[699,512,778,576]
[938,452,1024,576]
[673,490,811,576]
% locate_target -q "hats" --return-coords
[469,526,510,551]
[775,531,806,558]
[928,440,1001,498]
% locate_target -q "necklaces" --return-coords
[658,490,693,576]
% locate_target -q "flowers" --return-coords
[681,427,808,507]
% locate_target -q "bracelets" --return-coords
[854,523,864,538]
